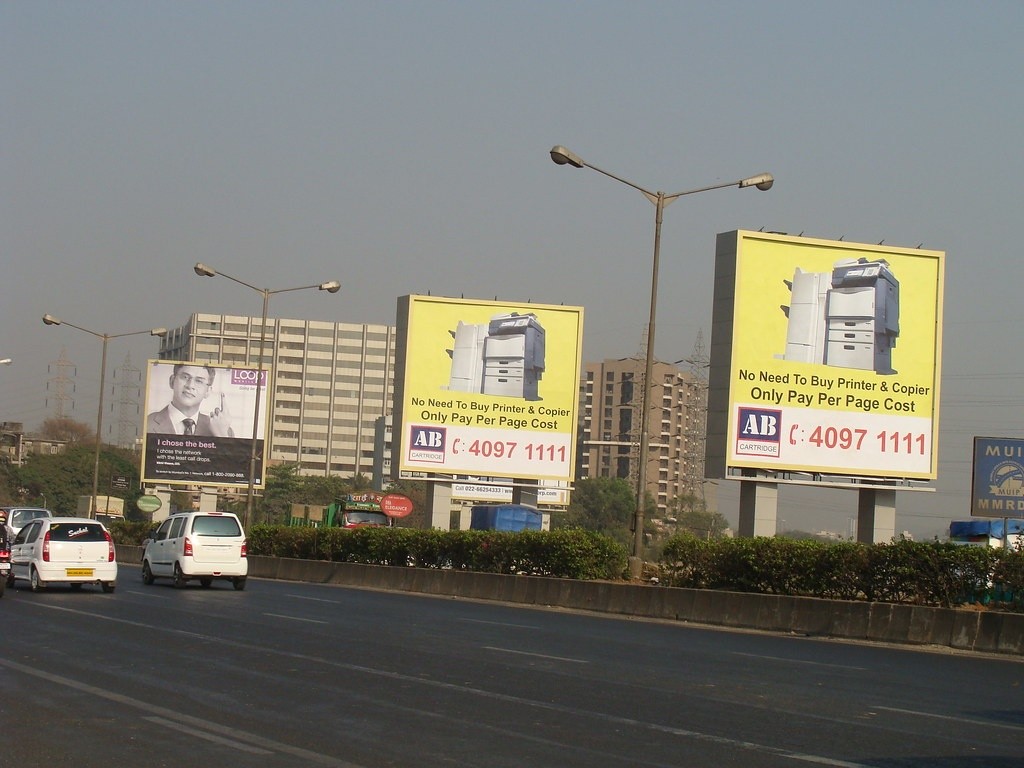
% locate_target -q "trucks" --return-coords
[76,494,127,535]
[322,500,393,531]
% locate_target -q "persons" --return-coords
[145,364,235,437]
[0,511,15,553]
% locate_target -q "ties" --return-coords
[183,419,195,435]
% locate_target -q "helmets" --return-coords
[0,511,7,525]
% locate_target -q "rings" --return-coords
[211,414,215,418]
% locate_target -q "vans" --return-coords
[141,510,250,590]
[0,505,52,552]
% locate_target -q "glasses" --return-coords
[181,373,208,385]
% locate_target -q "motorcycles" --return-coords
[0,540,14,600]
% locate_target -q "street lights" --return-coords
[41,314,167,527]
[548,146,776,562]
[193,261,341,541]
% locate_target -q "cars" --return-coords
[11,518,117,593]
